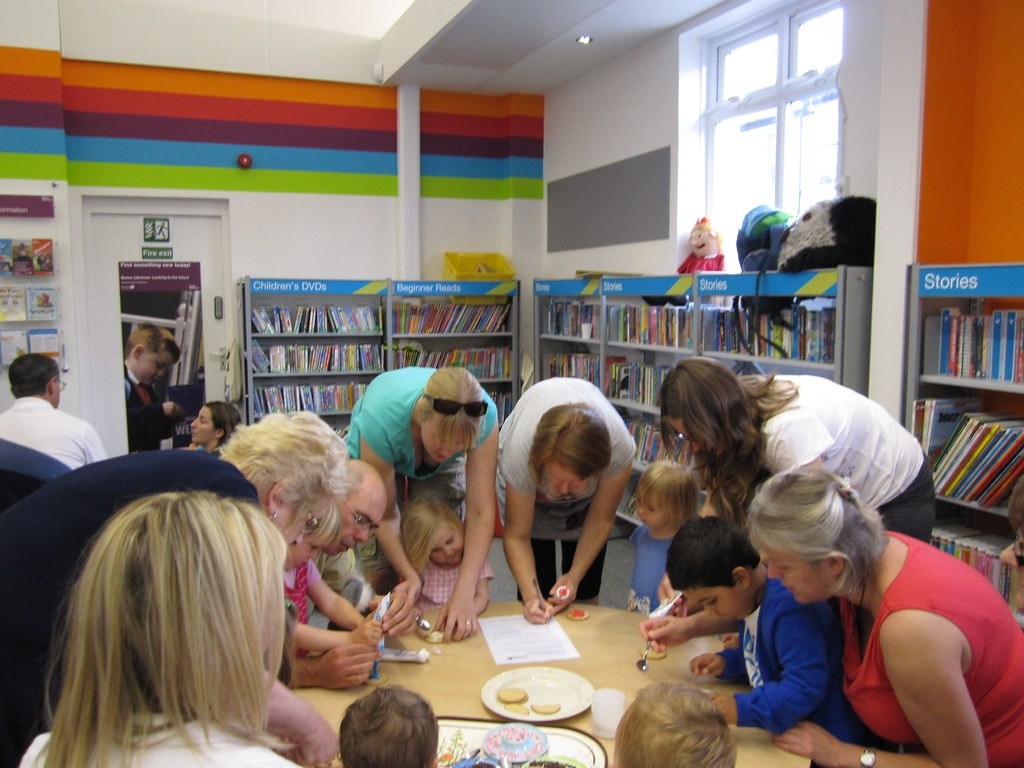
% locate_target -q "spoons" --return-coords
[414,616,430,630]
[636,639,653,672]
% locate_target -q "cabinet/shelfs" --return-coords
[244,276,393,439]
[532,274,605,394]
[388,279,521,433]
[899,260,1023,638]
[602,271,702,535]
[690,265,870,512]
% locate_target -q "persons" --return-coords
[744,467,1024,768]
[657,356,936,541]
[638,517,874,746]
[627,463,702,614]
[1,354,515,768]
[609,683,738,768]
[490,377,638,626]
[122,322,183,455]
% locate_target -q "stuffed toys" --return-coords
[677,217,725,272]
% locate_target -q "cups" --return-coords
[590,688,627,738]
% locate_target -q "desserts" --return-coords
[498,687,561,715]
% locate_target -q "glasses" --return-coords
[301,508,322,534]
[423,393,488,417]
[54,377,67,391]
[346,499,376,539]
[672,430,689,444]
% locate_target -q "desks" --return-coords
[292,598,816,768]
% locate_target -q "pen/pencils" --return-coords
[532,579,550,625]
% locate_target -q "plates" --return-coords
[482,666,595,722]
[438,715,609,768]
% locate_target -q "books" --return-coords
[906,306,1024,618]
[250,293,516,371]
[542,299,836,406]
[621,473,642,519]
[1,238,62,364]
[623,417,699,467]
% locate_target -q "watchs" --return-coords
[860,746,877,768]
